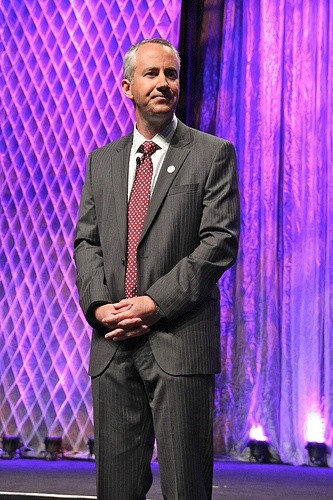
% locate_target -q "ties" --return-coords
[124,141,161,298]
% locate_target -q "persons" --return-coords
[73,39,241,500]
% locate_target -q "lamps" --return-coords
[1,436,22,457]
[247,439,270,463]
[304,441,328,467]
[40,436,66,460]
